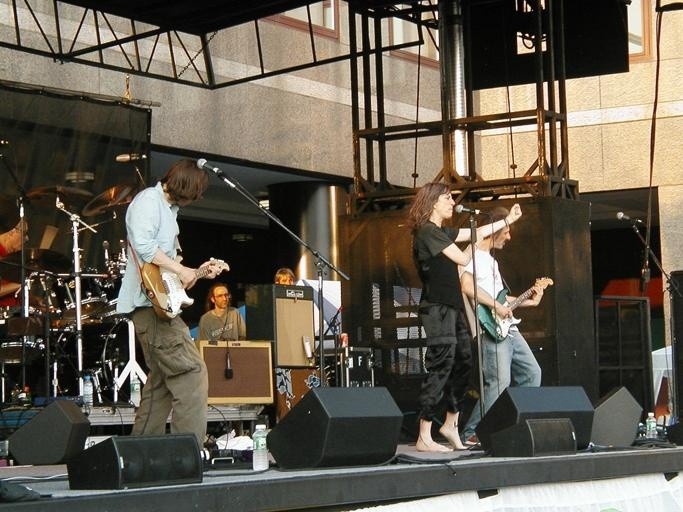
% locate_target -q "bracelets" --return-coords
[502,217,510,227]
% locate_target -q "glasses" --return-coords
[214,293,229,299]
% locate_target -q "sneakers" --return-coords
[465,436,481,446]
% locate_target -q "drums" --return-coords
[22,272,71,316]
[0,306,44,363]
[53,319,149,407]
[98,271,124,317]
[51,266,108,326]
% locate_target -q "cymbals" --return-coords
[21,185,93,205]
[81,182,136,217]
[16,247,73,280]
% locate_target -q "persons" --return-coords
[0,200,56,313]
[409,182,522,453]
[116,160,225,451]
[198,282,246,341]
[274,268,295,285]
[457,213,543,446]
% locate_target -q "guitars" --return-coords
[140,257,229,322]
[475,276,553,343]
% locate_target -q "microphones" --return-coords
[195,157,222,174]
[453,202,488,217]
[115,152,148,164]
[616,210,644,229]
[225,351,234,379]
[55,193,65,211]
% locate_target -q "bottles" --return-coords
[82,375,94,408]
[645,412,657,438]
[130,374,141,408]
[252,424,269,471]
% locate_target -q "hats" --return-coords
[0,200,21,235]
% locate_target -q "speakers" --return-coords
[270,385,405,470]
[474,383,644,456]
[5,399,92,466]
[195,338,274,404]
[245,284,315,368]
[65,432,204,490]
[666,270,683,441]
